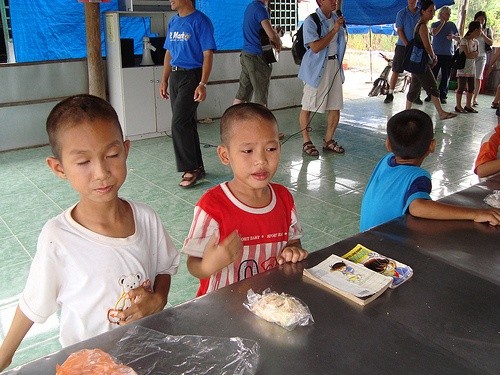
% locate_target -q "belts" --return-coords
[171,66,187,72]
[328,54,338,60]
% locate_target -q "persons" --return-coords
[360,109,500,234]
[181,102,308,299]
[490,28,500,109]
[424,7,460,104]
[233,0,285,140]
[159,0,218,189]
[474,103,500,178]
[0,94,180,375]
[405,0,458,120]
[463,11,494,106]
[455,21,483,114]
[299,0,349,157]
[383,0,435,103]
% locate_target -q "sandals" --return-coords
[322,138,345,153]
[197,169,206,180]
[491,102,499,108]
[302,140,319,157]
[179,166,201,187]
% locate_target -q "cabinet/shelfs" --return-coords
[103,10,174,141]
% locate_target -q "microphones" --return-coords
[336,9,346,28]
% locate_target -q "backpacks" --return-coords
[291,12,321,66]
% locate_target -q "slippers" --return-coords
[440,112,457,120]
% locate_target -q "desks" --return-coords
[2,173,500,375]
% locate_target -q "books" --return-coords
[303,244,414,305]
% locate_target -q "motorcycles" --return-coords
[368,52,412,97]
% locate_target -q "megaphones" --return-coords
[139,36,156,65]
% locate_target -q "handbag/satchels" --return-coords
[484,27,492,53]
[452,38,469,69]
[402,23,429,74]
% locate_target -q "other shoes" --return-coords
[413,97,423,105]
[455,105,467,113]
[441,98,446,104]
[278,133,284,140]
[464,106,478,113]
[473,102,478,107]
[384,93,393,103]
[424,95,432,102]
